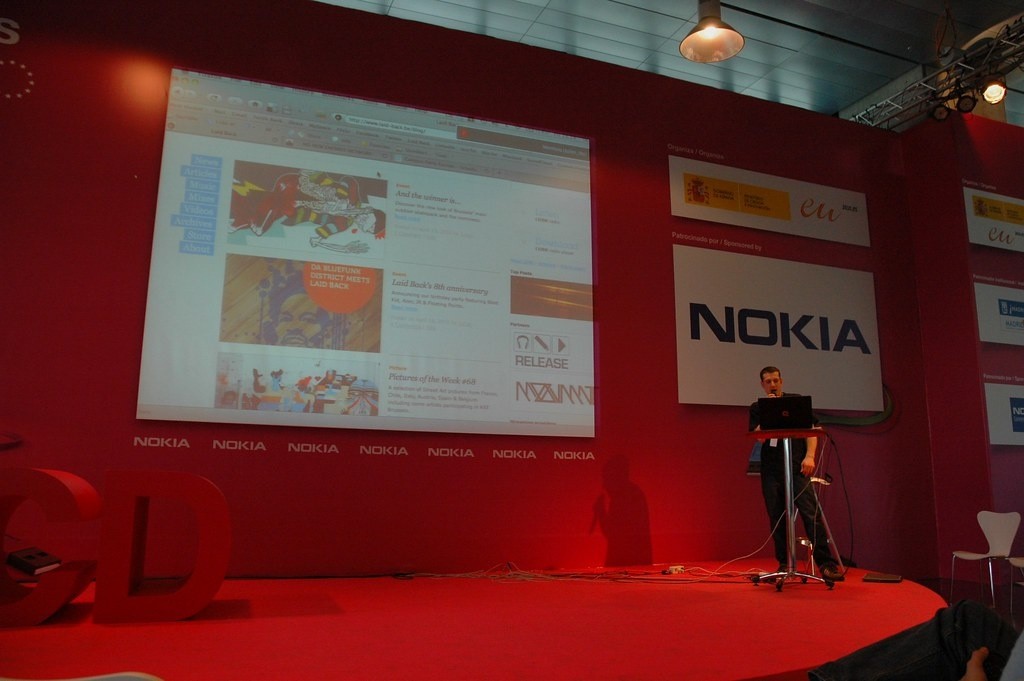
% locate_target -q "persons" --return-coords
[808,599,1024,681]
[746,368,846,580]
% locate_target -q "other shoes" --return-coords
[821,565,845,581]
[772,567,796,575]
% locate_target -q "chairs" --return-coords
[948,510,1021,613]
[1005,557,1024,618]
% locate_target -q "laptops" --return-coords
[757,396,812,428]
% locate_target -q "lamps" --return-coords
[680,1,745,64]
